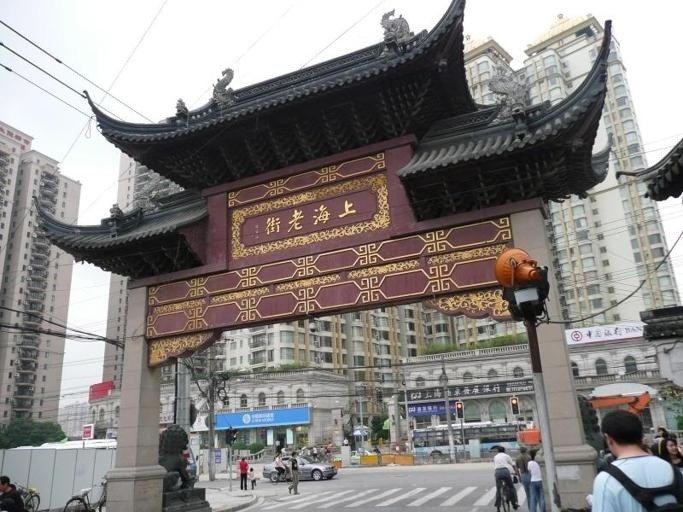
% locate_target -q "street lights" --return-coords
[494,247,562,511]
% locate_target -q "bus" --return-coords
[407,418,537,463]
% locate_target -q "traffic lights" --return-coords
[225,428,229,444]
[509,397,519,415]
[455,401,464,419]
[228,428,238,447]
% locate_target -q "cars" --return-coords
[261,452,337,482]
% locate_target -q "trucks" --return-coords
[184,447,198,489]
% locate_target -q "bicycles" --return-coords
[61,477,107,511]
[497,472,518,512]
[13,481,40,511]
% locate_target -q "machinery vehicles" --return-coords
[514,390,649,459]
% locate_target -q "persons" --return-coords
[323,445,331,463]
[493,446,520,511]
[287,451,299,494]
[239,457,248,490]
[372,445,381,454]
[246,467,256,489]
[312,444,320,461]
[0,475,25,511]
[582,407,682,511]
[272,444,281,459]
[274,438,280,447]
[514,445,536,511]
[272,451,285,478]
[526,449,546,511]
[342,436,348,445]
[318,445,325,457]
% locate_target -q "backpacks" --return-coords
[597,462,683,512]
